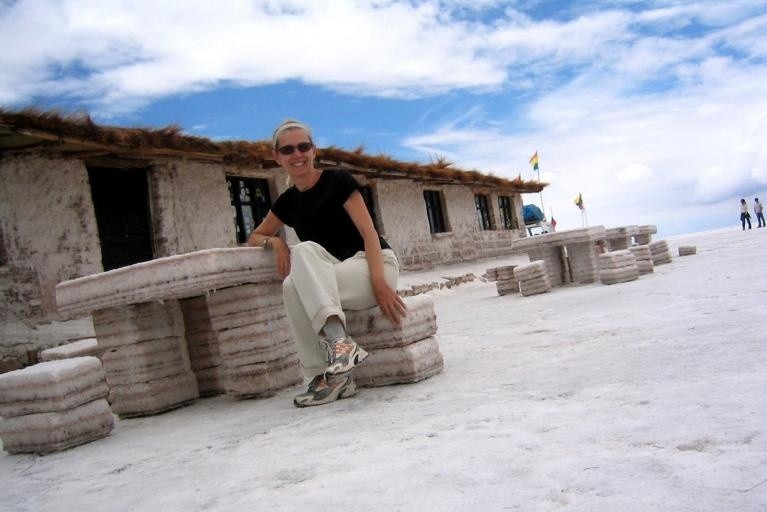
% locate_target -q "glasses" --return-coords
[276,143,313,155]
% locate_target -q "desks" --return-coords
[510,225,610,285]
[632,224,657,245]
[604,225,638,252]
[53,244,303,419]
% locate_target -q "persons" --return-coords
[754,198,766,227]
[249,120,406,406]
[741,199,751,229]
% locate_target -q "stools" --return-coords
[340,293,444,386]
[1,356,114,456]
[40,337,98,362]
[483,237,698,299]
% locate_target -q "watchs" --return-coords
[263,236,269,249]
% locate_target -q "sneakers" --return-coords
[318,334,369,375]
[293,367,360,406]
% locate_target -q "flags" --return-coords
[529,152,538,169]
[551,218,556,227]
[575,195,585,210]
